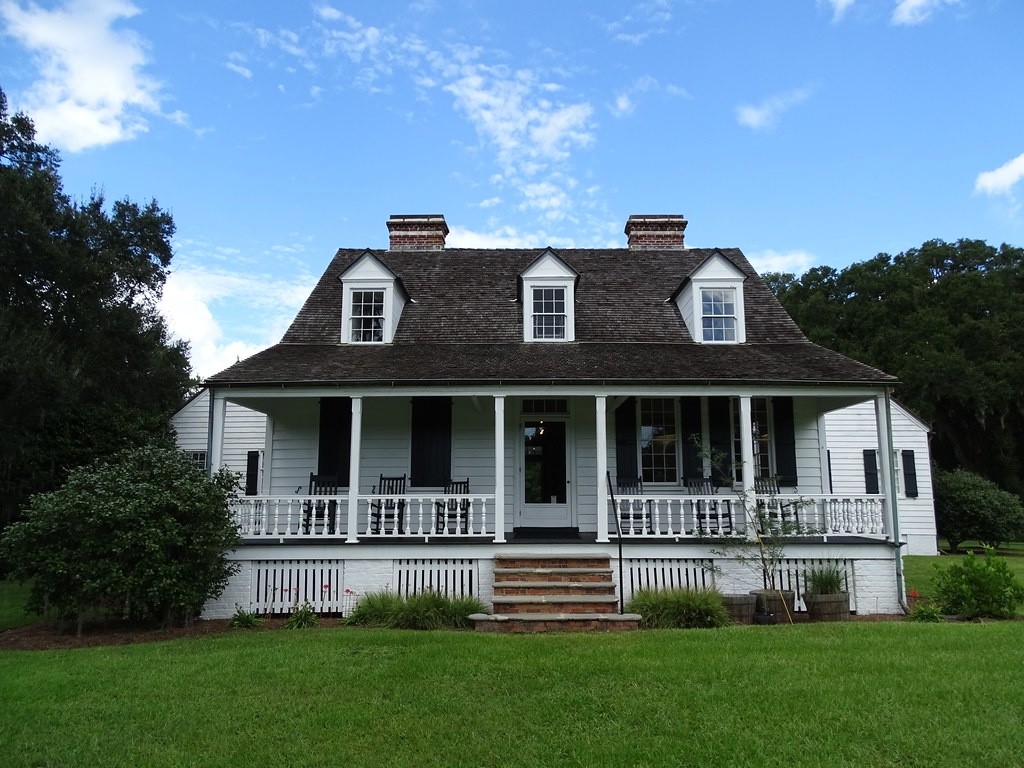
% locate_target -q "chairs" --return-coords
[606,471,654,536]
[368,473,408,534]
[434,479,471,536]
[755,476,801,536]
[688,478,733,535]
[300,472,336,535]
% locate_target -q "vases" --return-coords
[722,593,754,624]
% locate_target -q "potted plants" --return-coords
[801,559,852,623]
[687,434,797,623]
